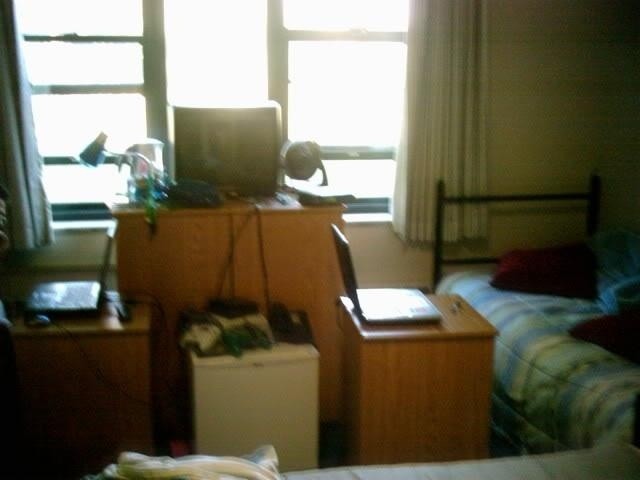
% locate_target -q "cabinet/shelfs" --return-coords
[187,340,323,471]
[10,295,152,472]
[331,290,499,465]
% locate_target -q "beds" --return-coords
[430,172,636,454]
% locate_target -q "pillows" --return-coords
[489,242,639,367]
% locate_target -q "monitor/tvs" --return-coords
[169,101,285,200]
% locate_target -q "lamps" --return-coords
[74,133,156,177]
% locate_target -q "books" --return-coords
[281,177,358,205]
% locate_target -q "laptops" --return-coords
[331,223,444,326]
[25,279,103,321]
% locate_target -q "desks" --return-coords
[110,197,350,429]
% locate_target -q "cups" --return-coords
[124,141,164,178]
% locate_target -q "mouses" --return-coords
[22,314,52,328]
